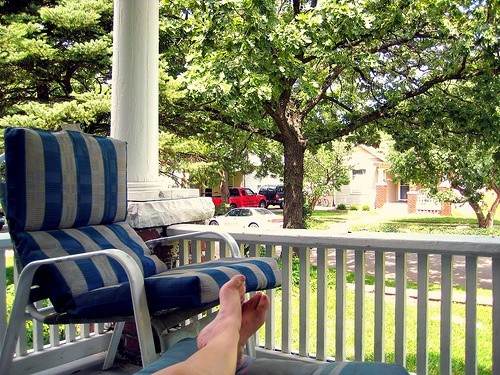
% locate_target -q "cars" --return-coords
[205,208,283,229]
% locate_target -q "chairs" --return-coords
[0,126,283,375]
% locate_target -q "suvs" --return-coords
[260,184,284,208]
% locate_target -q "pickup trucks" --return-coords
[213,187,268,208]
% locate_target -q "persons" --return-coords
[149,274,270,375]
